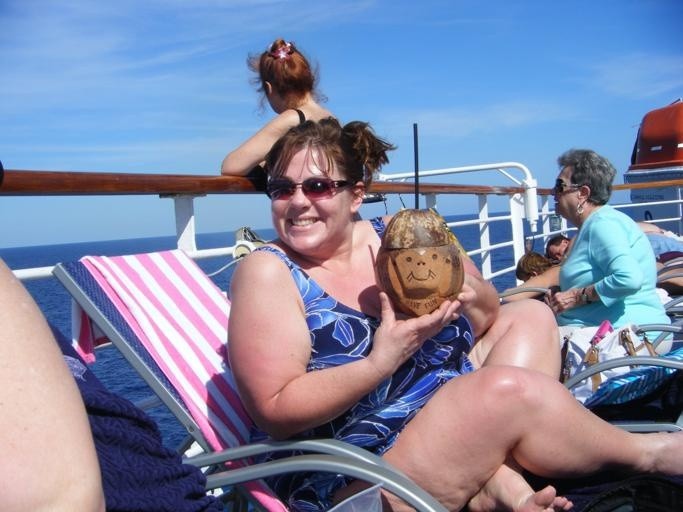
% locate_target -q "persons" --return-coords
[0,258,105,512]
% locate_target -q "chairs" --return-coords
[488,285,681,417]
[48,245,682,511]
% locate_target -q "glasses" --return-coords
[556,178,582,192]
[263,174,353,198]
